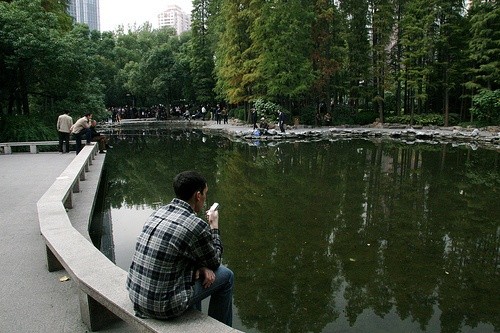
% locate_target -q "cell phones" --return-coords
[207,202,220,215]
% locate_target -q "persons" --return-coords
[315,96,360,123]
[126,170,235,326]
[107,103,230,125]
[70,113,108,155]
[249,107,269,136]
[275,109,286,133]
[57,109,73,153]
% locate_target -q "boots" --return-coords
[99,150,106,154]
[105,143,114,149]
